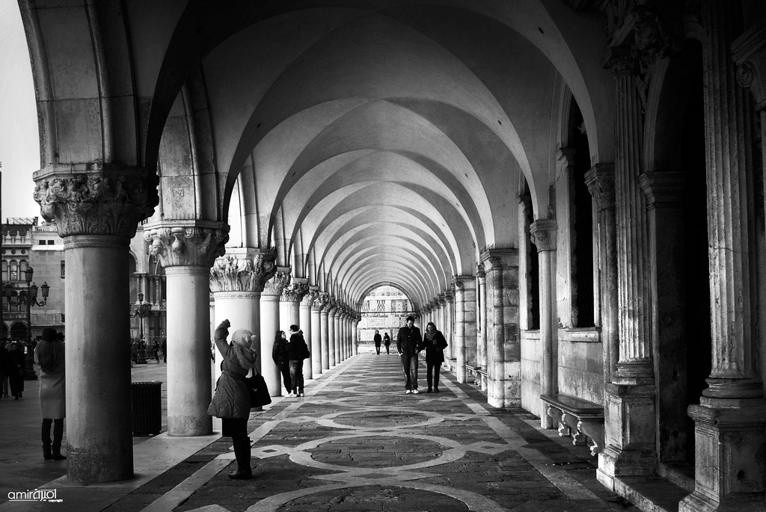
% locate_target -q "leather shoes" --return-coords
[427,388,433,393]
[434,388,439,393]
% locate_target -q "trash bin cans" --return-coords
[131,381,162,437]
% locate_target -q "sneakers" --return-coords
[405,390,411,394]
[411,389,419,394]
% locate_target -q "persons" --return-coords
[35,325,67,461]
[0,332,66,400]
[383,333,390,355]
[272,331,301,397]
[374,330,381,355]
[285,325,310,398]
[397,316,422,394]
[421,322,448,392]
[130,336,167,365]
[206,319,259,480]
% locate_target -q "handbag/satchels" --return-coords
[246,366,271,408]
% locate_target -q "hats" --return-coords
[56,332,65,341]
[40,327,58,341]
[231,329,256,349]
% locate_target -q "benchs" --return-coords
[540,394,603,458]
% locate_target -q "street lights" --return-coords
[128,292,151,364]
[4,265,48,381]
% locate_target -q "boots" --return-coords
[227,437,254,480]
[42,446,53,460]
[51,446,67,460]
